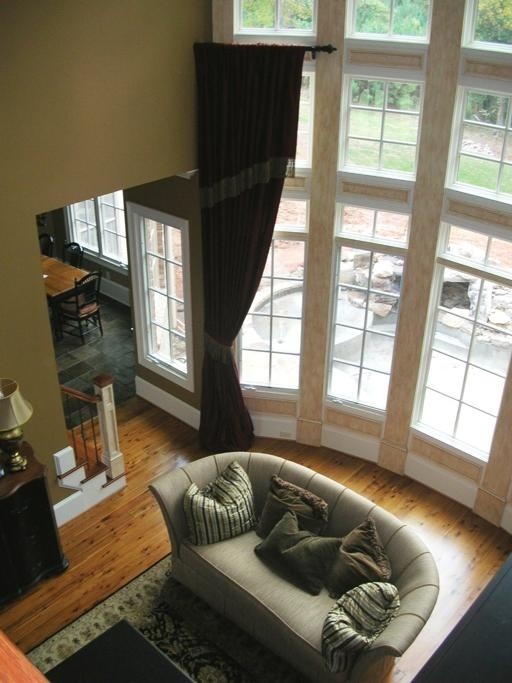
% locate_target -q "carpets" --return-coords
[24,552,316,683]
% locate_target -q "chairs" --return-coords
[39,233,54,258]
[55,269,105,345]
[61,242,83,270]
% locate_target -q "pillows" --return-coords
[183,459,257,548]
[320,583,401,674]
[259,473,327,542]
[330,517,394,599]
[255,512,345,597]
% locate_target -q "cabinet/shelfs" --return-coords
[0,441,70,611]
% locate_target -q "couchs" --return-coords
[145,450,441,683]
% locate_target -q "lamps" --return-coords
[1,377,35,472]
